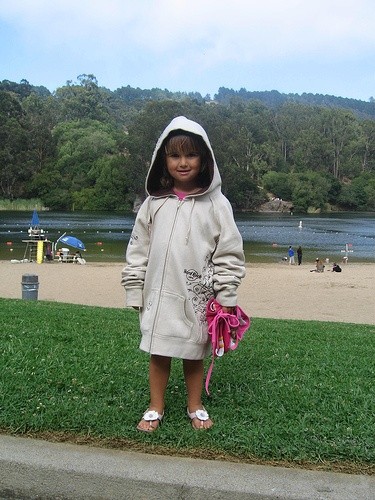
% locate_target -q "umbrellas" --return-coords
[59,236,86,251]
[31,208,40,230]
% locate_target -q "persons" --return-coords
[75,251,82,264]
[282,245,342,273]
[121,118,245,433]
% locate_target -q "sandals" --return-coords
[187,405,213,431]
[137,407,164,432]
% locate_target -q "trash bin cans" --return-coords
[20,274,39,301]
